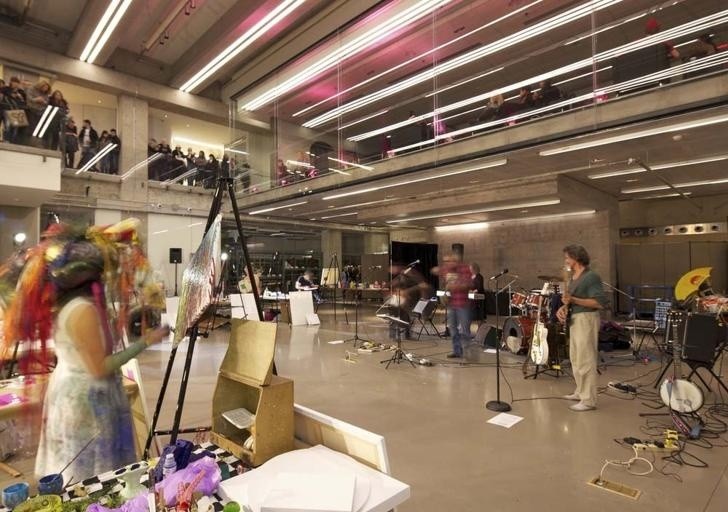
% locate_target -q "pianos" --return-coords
[435,289,486,301]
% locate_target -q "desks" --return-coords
[0,438,411,512]
[0,373,142,463]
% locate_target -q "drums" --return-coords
[509,290,528,311]
[504,314,534,355]
[527,287,549,311]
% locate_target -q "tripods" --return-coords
[385,279,415,368]
[524,290,573,382]
[315,253,349,324]
[344,284,368,347]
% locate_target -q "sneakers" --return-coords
[569,403,596,411]
[564,393,580,400]
[448,352,462,358]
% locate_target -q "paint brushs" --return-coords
[51,429,102,480]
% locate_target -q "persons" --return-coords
[645,23,681,58]
[296,270,327,306]
[555,245,604,412]
[697,32,718,59]
[469,262,487,325]
[0,75,120,177]
[36,243,171,487]
[432,244,475,360]
[148,137,234,188]
[478,80,565,127]
[276,152,320,183]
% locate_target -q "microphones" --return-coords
[490,268,509,282]
[408,259,420,267]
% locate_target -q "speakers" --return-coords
[664,311,717,364]
[170,247,182,266]
[411,297,434,320]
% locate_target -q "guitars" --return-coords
[530,282,550,366]
[559,260,570,347]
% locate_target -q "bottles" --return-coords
[162,453,177,481]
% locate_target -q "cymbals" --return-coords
[537,275,564,283]
[507,271,530,283]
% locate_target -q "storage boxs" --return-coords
[210,318,294,467]
